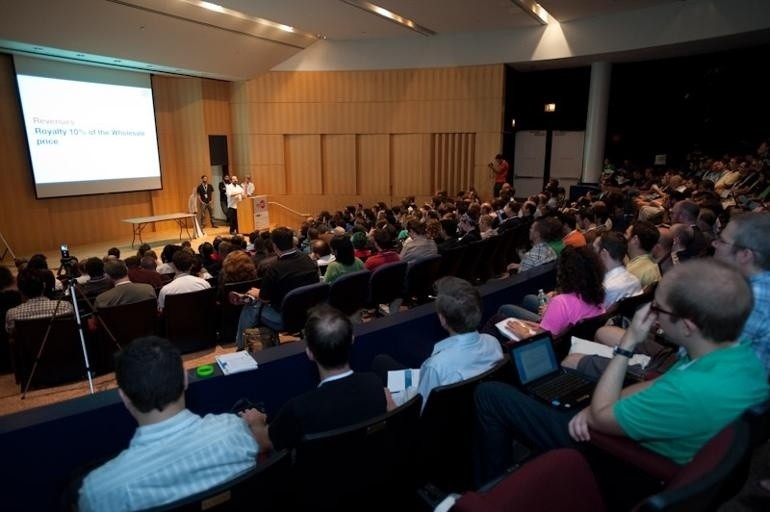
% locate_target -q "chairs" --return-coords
[2,222,769,512]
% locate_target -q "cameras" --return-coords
[488,162,493,166]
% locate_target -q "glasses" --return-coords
[650,301,674,315]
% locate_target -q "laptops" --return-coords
[510,330,598,412]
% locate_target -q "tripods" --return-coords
[21,255,122,401]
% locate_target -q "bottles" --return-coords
[537,289,548,305]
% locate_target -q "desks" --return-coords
[121,212,197,248]
